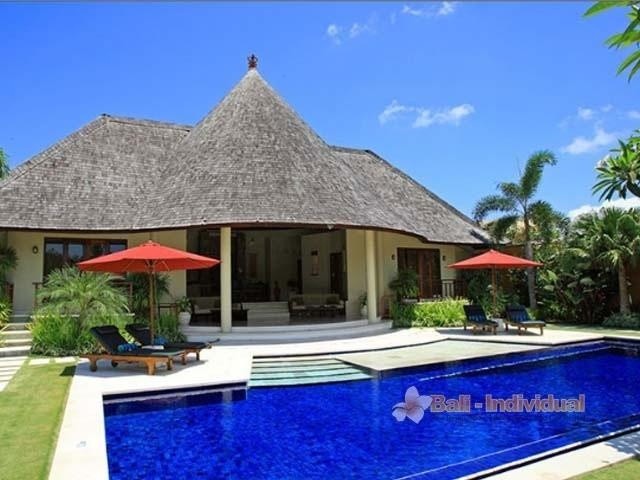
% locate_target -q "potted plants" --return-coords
[175,294,195,325]
[391,268,420,306]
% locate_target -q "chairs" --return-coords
[287,293,344,319]
[79,323,213,376]
[461,301,546,338]
